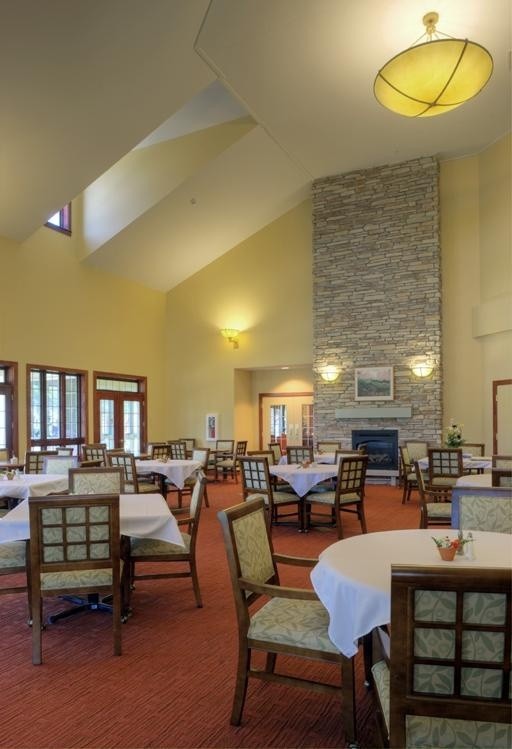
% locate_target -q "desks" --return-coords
[319,528,509,604]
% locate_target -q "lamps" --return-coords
[318,366,341,382]
[408,363,435,379]
[372,11,497,118]
[219,327,238,349]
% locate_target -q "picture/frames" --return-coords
[205,413,218,442]
[354,366,396,401]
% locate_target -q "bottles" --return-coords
[456,531,464,556]
[465,534,476,563]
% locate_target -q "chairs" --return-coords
[167,438,511,540]
[372,563,511,748]
[0,433,211,666]
[216,497,373,746]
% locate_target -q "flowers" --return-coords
[443,418,466,449]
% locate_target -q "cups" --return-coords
[302,464,308,469]
[438,546,455,561]
